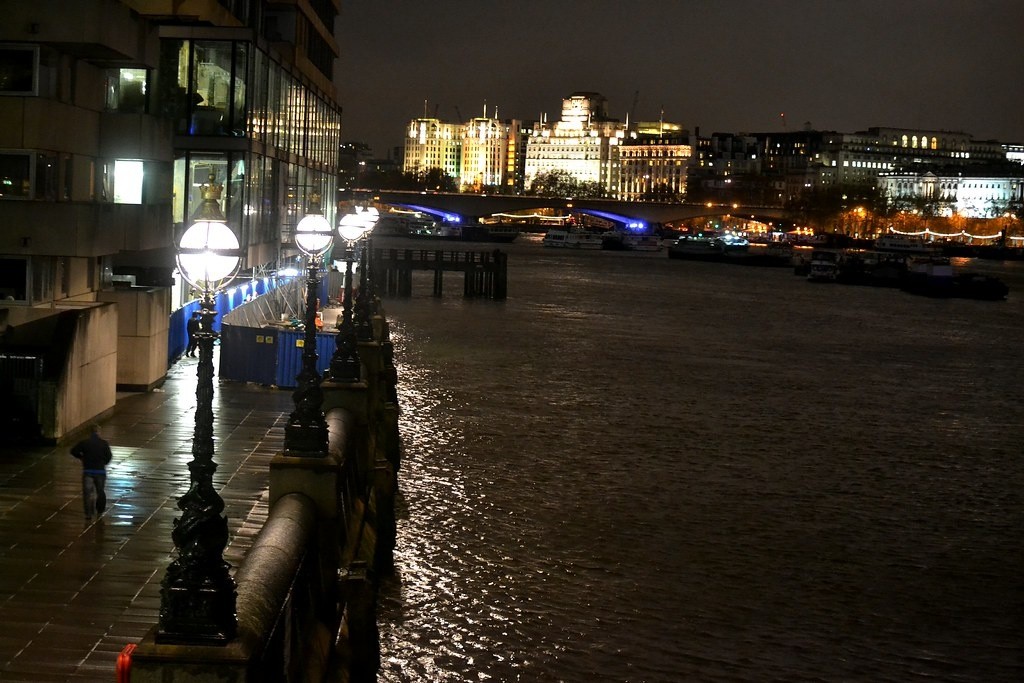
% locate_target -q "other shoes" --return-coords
[186,353,189,357]
[191,353,196,358]
[86,516,92,520]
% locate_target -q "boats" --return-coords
[543,230,603,250]
[875,233,943,258]
[602,233,662,252]
[812,247,852,281]
[769,237,799,258]
[670,235,727,255]
[408,224,461,241]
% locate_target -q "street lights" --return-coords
[329,208,364,383]
[152,171,238,645]
[356,203,380,343]
[284,185,335,461]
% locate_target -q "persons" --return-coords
[70,425,113,520]
[186,310,201,356]
[332,265,339,271]
